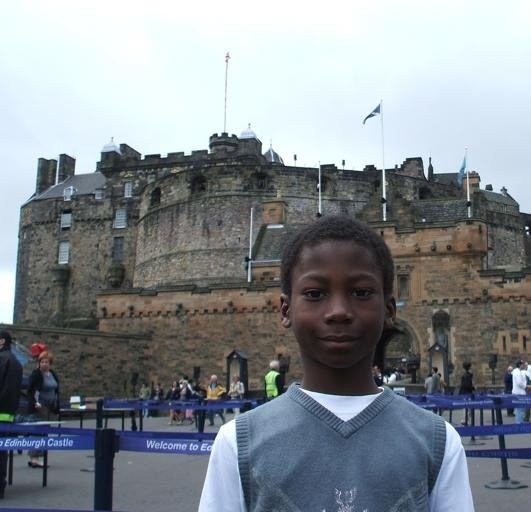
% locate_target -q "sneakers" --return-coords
[28,460,50,469]
[141,415,227,427]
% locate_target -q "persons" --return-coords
[0,332,23,498]
[26,351,60,468]
[197,218,474,512]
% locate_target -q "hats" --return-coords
[515,359,525,364]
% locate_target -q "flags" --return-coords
[362,104,380,123]
[457,158,466,187]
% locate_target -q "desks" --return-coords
[57,405,135,434]
